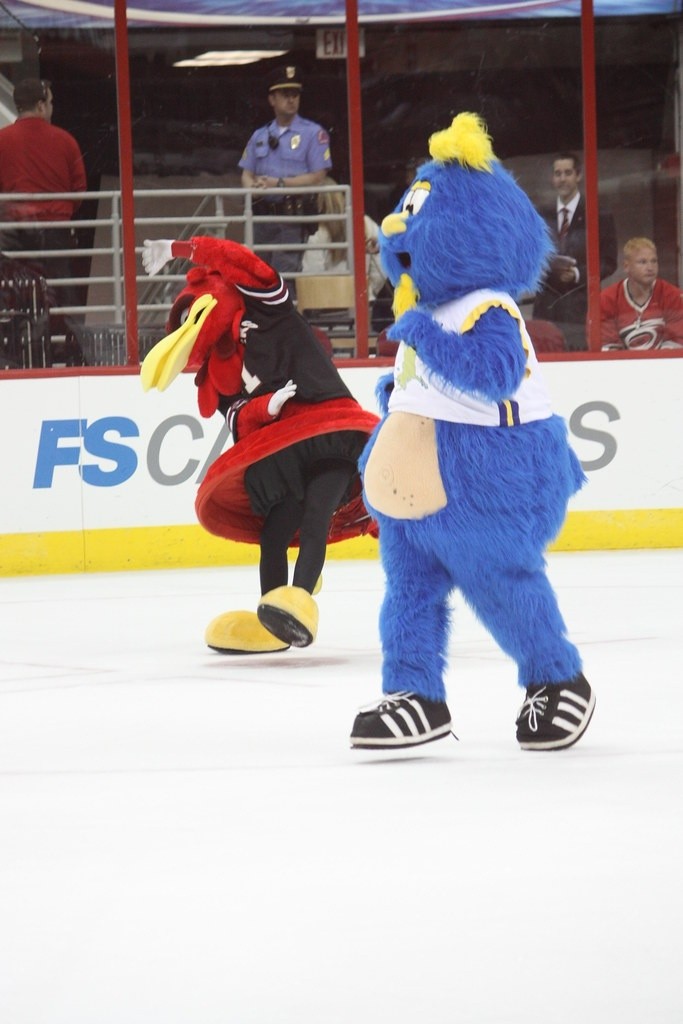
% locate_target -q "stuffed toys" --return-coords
[350,117,595,750]
[141,230,382,653]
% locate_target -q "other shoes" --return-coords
[256,586,319,648]
[205,611,290,654]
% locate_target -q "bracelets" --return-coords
[277,176,285,189]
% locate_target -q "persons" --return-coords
[0,78,85,306]
[300,157,430,332]
[587,236,683,352]
[528,151,619,353]
[238,67,332,297]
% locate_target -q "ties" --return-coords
[559,209,569,248]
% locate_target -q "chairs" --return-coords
[296,274,378,356]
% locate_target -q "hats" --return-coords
[265,63,308,93]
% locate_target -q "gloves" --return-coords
[392,273,417,323]
[141,239,176,278]
[268,380,297,419]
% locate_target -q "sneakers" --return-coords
[350,691,451,745]
[515,672,596,750]
